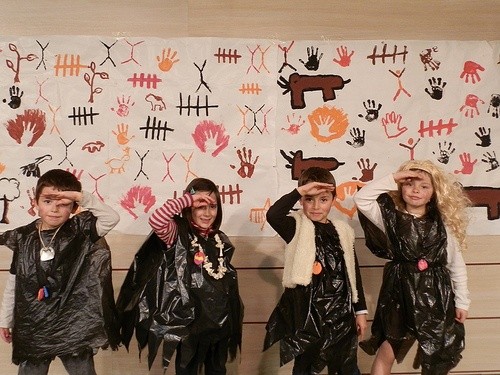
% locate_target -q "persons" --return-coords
[262,167,369,374]
[0,169,120,375]
[116,178,245,375]
[355,159,474,375]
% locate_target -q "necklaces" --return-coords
[36,216,65,261]
